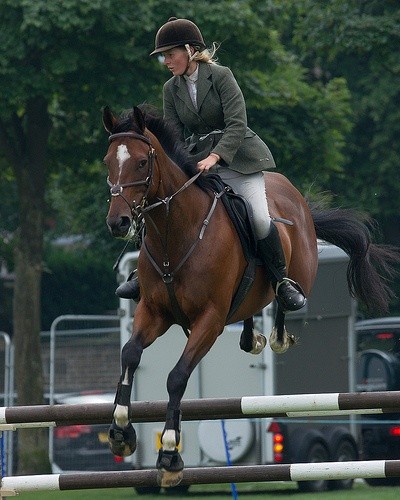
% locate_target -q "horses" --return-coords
[101,99,397,489]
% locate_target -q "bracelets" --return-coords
[210,152,220,159]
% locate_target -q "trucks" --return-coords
[118,239,400,492]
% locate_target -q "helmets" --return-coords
[149,17,206,56]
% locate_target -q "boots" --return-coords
[257,220,306,312]
[114,276,140,298]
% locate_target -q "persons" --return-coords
[116,17,307,311]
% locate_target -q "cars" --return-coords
[41,390,125,472]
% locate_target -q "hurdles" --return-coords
[0,391,400,500]
